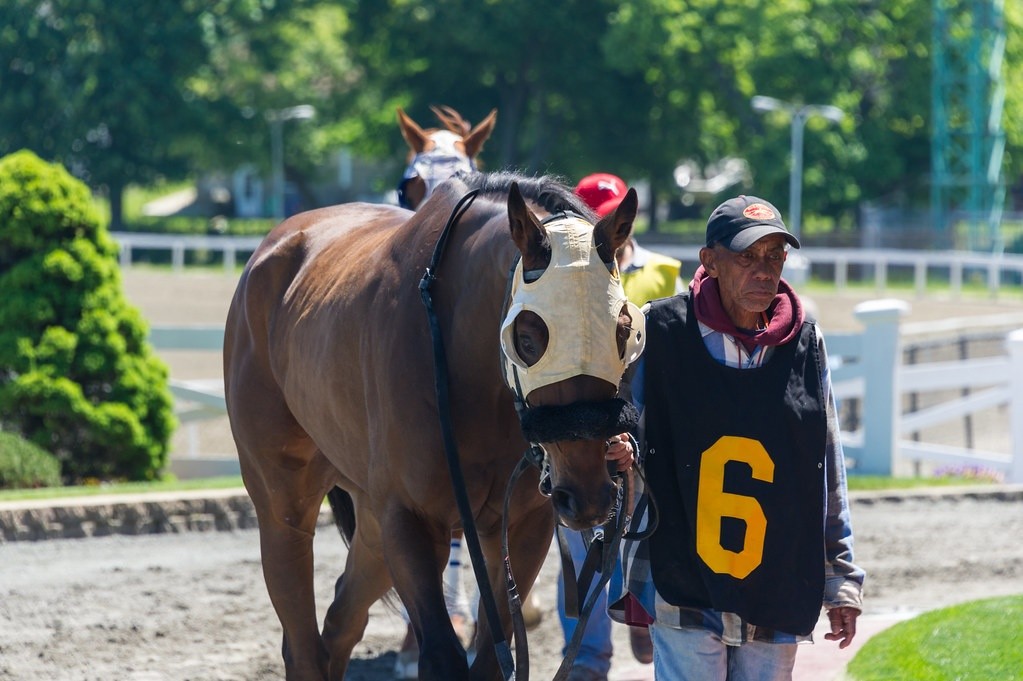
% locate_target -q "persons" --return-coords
[554,175,683,680]
[603,196,865,681]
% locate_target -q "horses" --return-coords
[222,165,640,679]
[394,105,500,212]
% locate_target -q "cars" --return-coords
[145,184,235,220]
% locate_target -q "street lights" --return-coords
[267,103,316,217]
[751,91,848,244]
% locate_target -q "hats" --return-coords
[705,194,800,251]
[575,173,629,219]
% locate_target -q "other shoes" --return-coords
[565,664,608,681]
[629,625,653,663]
